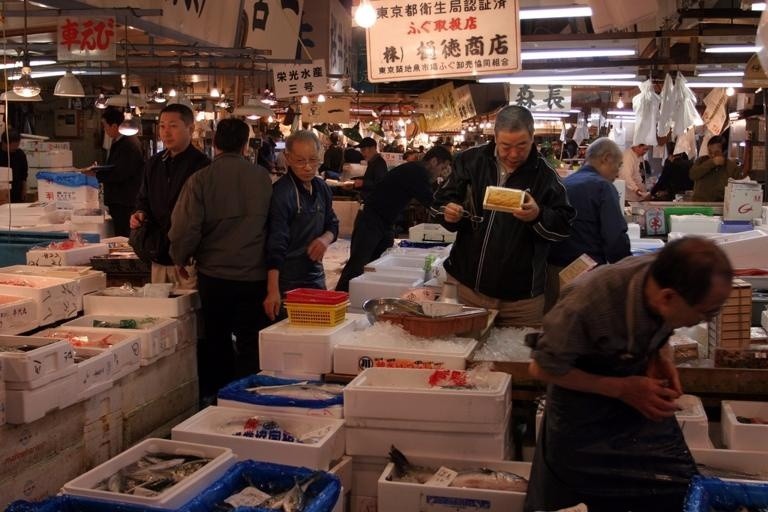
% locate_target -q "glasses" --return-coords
[696,300,730,323]
[289,154,323,168]
[459,209,484,223]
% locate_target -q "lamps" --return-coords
[0,0,768,138]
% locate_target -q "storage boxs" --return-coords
[0,113,767,512]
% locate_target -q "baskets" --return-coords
[282,288,351,327]
[381,303,492,338]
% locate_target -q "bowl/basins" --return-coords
[362,297,423,327]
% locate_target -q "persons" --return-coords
[522,236,734,511]
[0,129,28,204]
[166,116,273,410]
[129,103,212,283]
[80,106,145,268]
